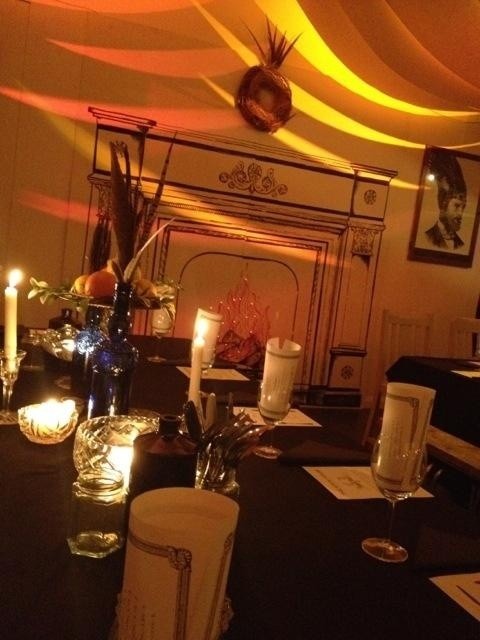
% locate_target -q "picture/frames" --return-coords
[408,145,480,267]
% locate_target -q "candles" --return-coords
[189,321,204,410]
[4,268,22,375]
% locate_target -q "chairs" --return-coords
[362,309,434,448]
[449,318,480,360]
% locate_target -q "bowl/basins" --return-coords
[15,405,77,444]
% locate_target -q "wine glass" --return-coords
[362,438,428,563]
[0,350,26,425]
[199,348,216,399]
[253,385,291,458]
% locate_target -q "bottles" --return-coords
[45,282,196,559]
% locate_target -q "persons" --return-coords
[422,152,469,250]
